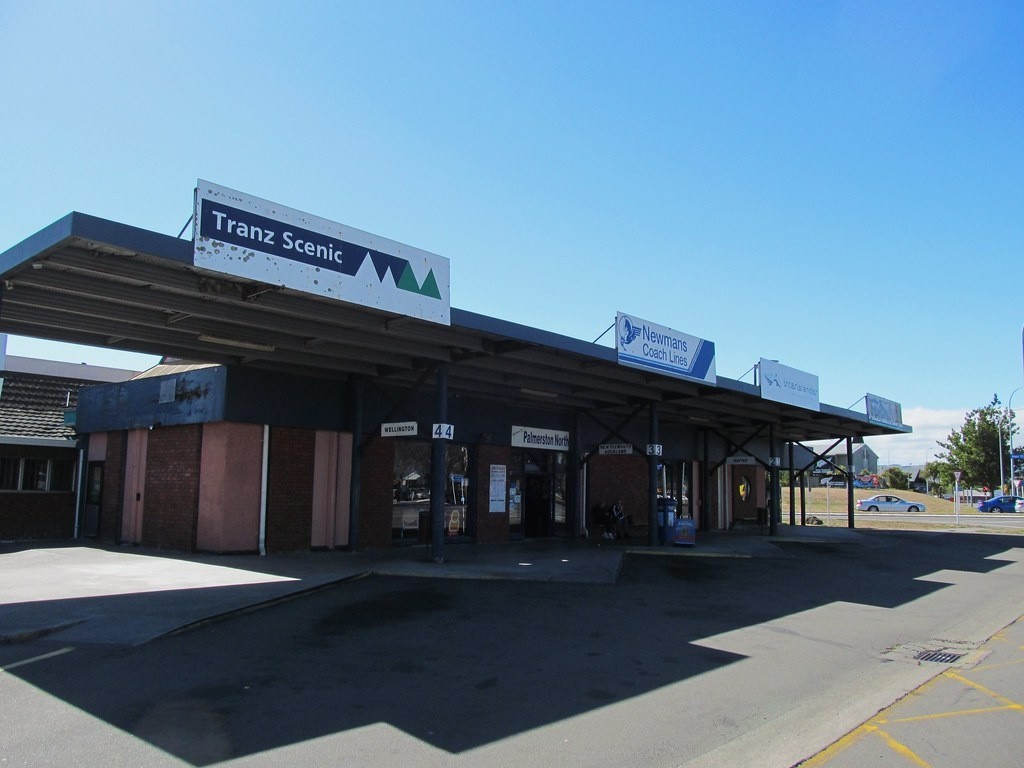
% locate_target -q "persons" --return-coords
[596,498,624,539]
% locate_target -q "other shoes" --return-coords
[608,534,614,539]
[602,534,610,539]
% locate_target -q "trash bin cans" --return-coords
[658,497,678,546]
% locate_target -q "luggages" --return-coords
[672,514,695,548]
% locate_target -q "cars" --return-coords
[977,495,1024,514]
[855,494,926,513]
[1014,499,1024,512]
